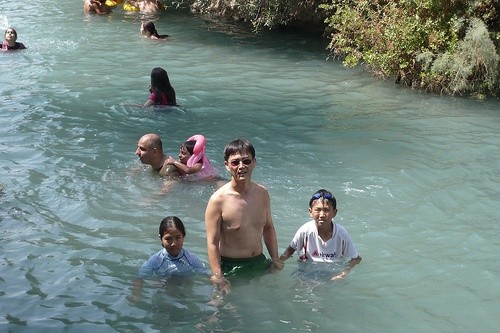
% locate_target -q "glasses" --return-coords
[231,159,251,166]
[311,192,332,199]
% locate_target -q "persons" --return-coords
[280,189,363,287]
[131,216,231,303]
[127,133,231,211]
[83,0,160,19]
[118,67,176,113]
[0,28,27,51]
[139,20,170,39]
[204,139,284,286]
